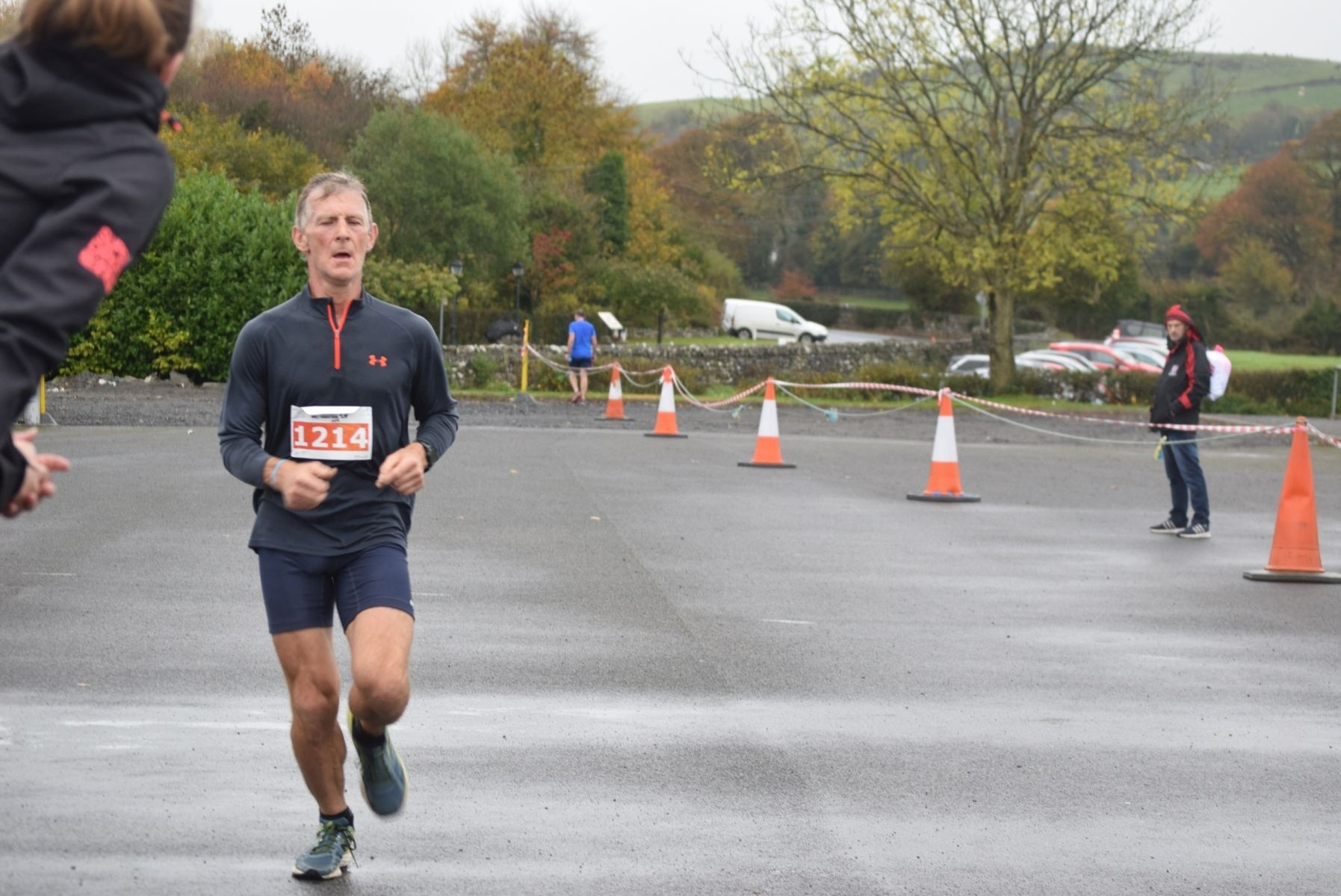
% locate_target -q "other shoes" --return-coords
[572,392,580,403]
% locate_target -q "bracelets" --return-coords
[271,459,288,490]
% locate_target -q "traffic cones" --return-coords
[594,362,634,423]
[904,387,982,503]
[736,377,797,468]
[1244,416,1341,584]
[643,365,688,438]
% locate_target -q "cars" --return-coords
[944,319,1177,409]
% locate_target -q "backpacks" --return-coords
[1184,341,1232,401]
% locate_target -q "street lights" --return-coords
[511,258,524,346]
[451,253,463,349]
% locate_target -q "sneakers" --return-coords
[293,815,360,880]
[1177,519,1211,538]
[347,707,406,819]
[1150,515,1187,533]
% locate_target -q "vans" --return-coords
[719,298,829,346]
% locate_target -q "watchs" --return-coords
[421,441,436,472]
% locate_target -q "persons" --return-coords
[564,309,597,406]
[0,0,198,519]
[1147,303,1212,539]
[218,172,461,884]
